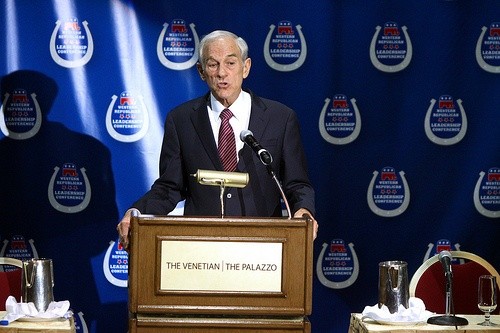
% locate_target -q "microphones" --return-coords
[240,130,273,165]
[438,249,453,288]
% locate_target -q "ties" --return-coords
[217,108,238,173]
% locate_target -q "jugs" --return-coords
[377,260,409,314]
[23,257,54,310]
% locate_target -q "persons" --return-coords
[117,31,318,249]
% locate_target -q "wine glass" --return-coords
[476,274,500,328]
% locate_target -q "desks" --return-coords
[348,313,500,333]
[0,310,75,333]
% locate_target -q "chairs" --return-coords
[0,257,27,311]
[408,251,500,315]
